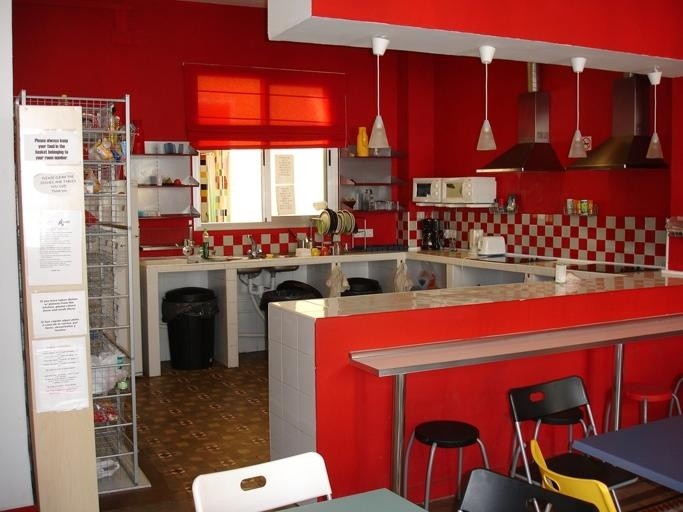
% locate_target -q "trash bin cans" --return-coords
[341,278,381,296]
[162,287,218,370]
[262,280,323,360]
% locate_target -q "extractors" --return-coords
[473,59,562,175]
[566,71,666,173]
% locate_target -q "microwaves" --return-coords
[442,176,497,207]
[412,177,442,207]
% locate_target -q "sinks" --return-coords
[209,258,262,275]
[265,253,299,272]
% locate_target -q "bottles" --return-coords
[357,126,370,157]
[133,119,145,155]
[202,228,210,260]
[297,239,342,256]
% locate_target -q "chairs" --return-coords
[508,376,639,512]
[192,452,333,512]
[531,440,617,512]
[459,468,600,512]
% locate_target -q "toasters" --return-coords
[477,236,505,256]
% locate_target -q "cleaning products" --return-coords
[202,228,209,259]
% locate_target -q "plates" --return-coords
[317,209,356,236]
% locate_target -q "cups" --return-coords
[469,229,484,252]
[554,284,566,297]
[555,262,566,284]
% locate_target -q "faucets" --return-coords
[246,235,257,259]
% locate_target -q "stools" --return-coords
[605,382,682,432]
[512,407,588,463]
[403,421,490,511]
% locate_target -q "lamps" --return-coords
[646,67,664,158]
[477,45,496,150]
[568,57,587,158]
[368,37,389,148]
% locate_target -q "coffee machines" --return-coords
[421,218,443,250]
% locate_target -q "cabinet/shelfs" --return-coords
[131,140,200,257]
[13,90,139,487]
[0,0,35,512]
[338,144,407,248]
[348,313,683,496]
